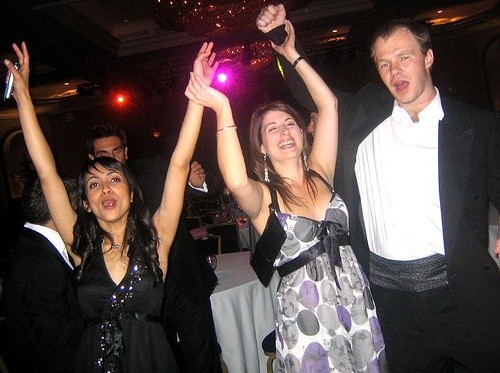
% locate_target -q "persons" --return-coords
[254,3,500,372]
[3,40,220,373]
[1,57,345,203]
[84,122,222,372]
[184,19,391,372]
[0,173,91,373]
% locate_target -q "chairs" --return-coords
[261,329,276,373]
[184,199,240,255]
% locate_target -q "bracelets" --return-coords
[292,56,304,68]
[215,124,238,135]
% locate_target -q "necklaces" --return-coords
[101,229,130,249]
[396,86,436,117]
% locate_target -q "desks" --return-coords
[209,249,282,373]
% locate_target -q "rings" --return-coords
[204,57,208,61]
